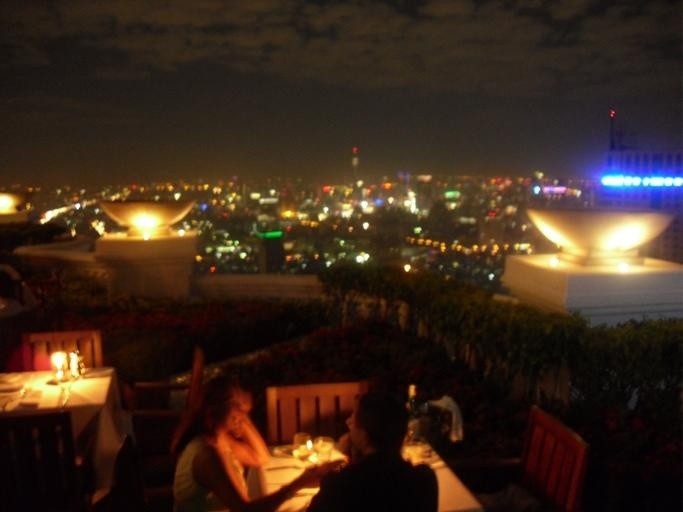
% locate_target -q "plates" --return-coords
[0,380,25,393]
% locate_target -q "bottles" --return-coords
[69,350,87,377]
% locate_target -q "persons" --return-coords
[302,386,439,509]
[0,263,36,318]
[169,371,344,511]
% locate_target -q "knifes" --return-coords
[292,492,316,498]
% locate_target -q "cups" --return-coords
[312,434,334,465]
[290,431,314,463]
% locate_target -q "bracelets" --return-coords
[13,273,23,287]
[278,483,297,500]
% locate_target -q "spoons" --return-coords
[266,465,304,471]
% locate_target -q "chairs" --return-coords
[20,329,104,476]
[135,343,208,473]
